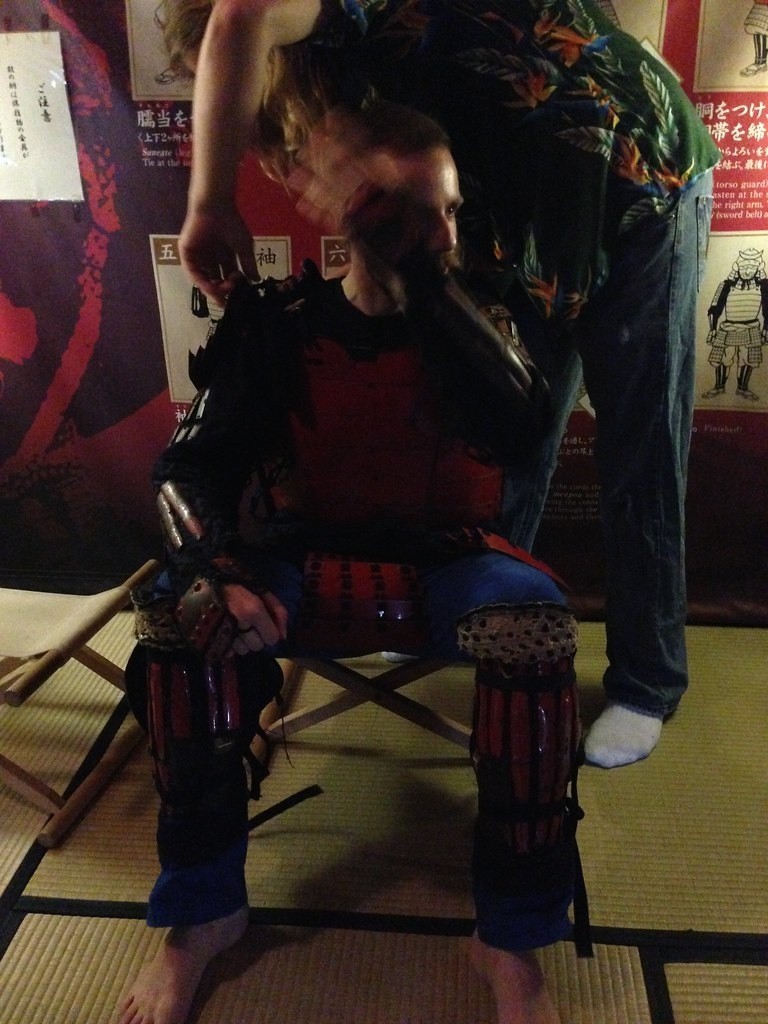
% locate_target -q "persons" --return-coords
[118,107,590,1024]
[165,0,724,768]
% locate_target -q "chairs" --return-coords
[183,273,551,773]
